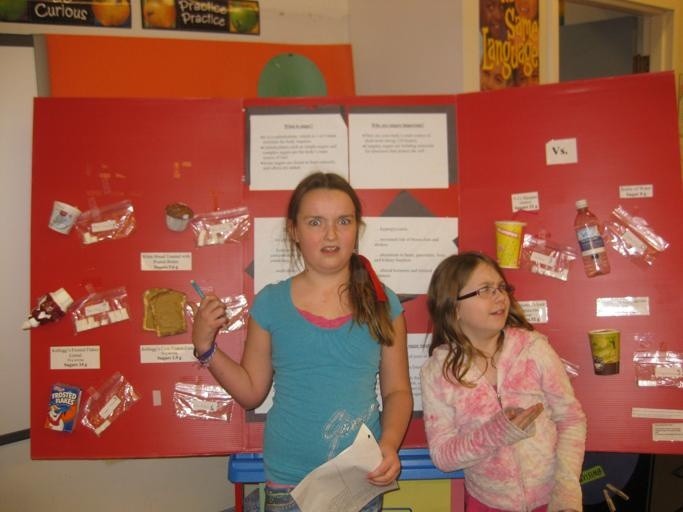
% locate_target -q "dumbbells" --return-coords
[257,52,327,98]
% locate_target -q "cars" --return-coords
[48,200,81,235]
[29,288,74,328]
[496,219,527,270]
[587,329,619,375]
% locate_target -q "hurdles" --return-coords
[231,7,256,32]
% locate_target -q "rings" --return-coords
[190,279,228,331]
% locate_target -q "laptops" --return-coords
[143,287,187,338]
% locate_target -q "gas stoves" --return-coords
[165,201,195,232]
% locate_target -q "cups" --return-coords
[455,282,515,301]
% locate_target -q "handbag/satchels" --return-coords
[196,344,217,364]
[193,342,215,360]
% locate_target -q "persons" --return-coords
[184,170,412,510]
[419,249,589,511]
[479,0,539,93]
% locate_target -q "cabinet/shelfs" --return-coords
[574,200,612,278]
